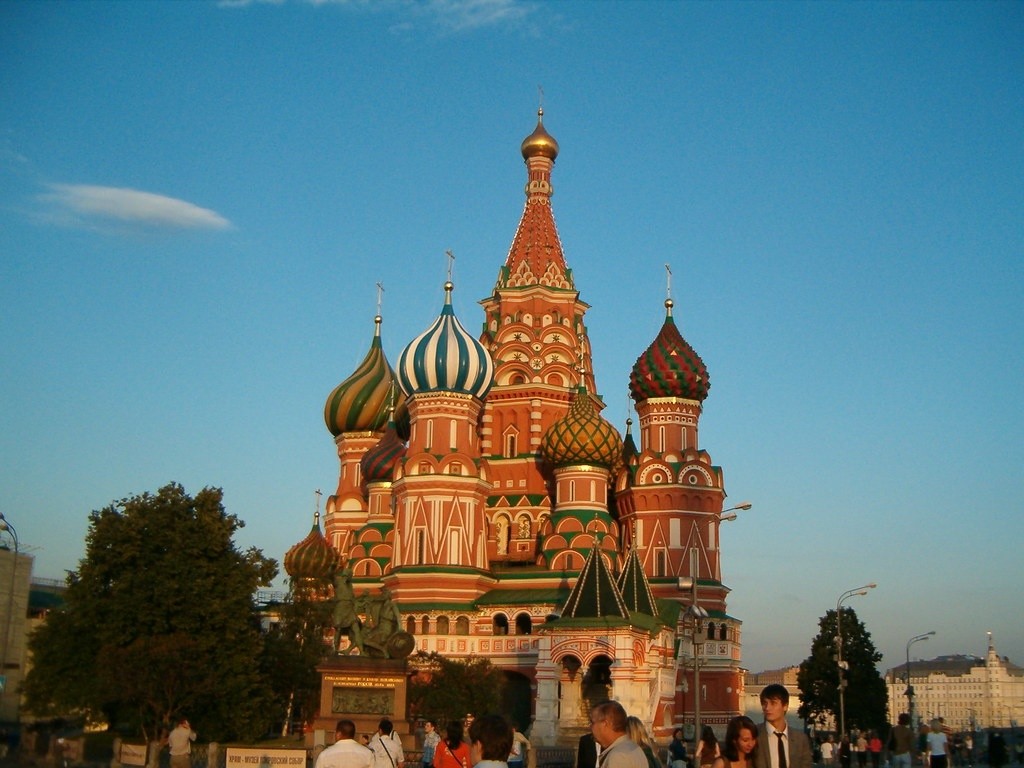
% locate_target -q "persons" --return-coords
[588,700,649,768]
[468,715,514,768]
[169,717,197,768]
[316,712,1024,768]
[754,684,811,768]
[331,569,402,658]
[711,716,759,768]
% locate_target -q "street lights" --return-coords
[927,711,934,721]
[903,687,916,733]
[906,631,937,688]
[0,512,18,694]
[837,582,877,740]
[690,502,752,768]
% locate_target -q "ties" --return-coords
[774,731,787,768]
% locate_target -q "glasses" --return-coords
[590,719,606,725]
